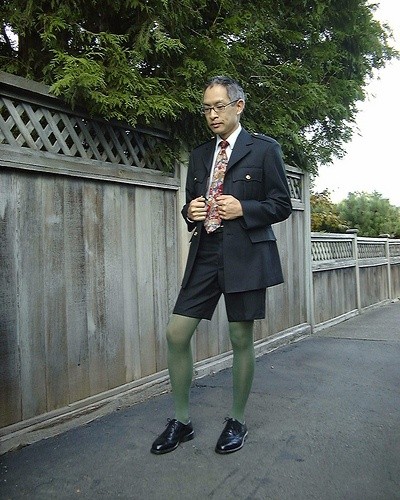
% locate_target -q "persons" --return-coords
[151,75,293,455]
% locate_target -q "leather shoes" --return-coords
[215,416,250,454]
[150,416,195,454]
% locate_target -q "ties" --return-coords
[203,141,231,234]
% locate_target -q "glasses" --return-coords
[201,99,238,113]
